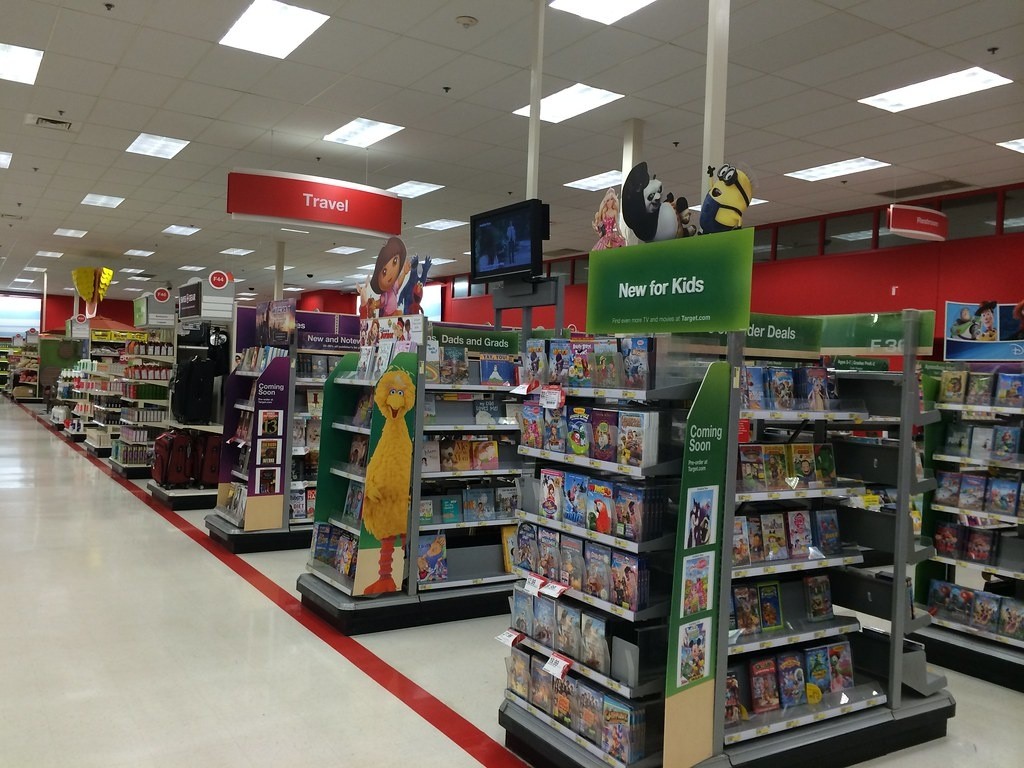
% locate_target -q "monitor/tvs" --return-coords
[469,198,550,284]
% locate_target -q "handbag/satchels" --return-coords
[177,320,212,345]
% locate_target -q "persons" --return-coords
[506,220,516,265]
[476,228,499,272]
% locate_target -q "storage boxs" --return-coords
[290,451,320,520]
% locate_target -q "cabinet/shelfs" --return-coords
[296,314,571,636]
[913,360,1024,693]
[0,332,232,513]
[203,345,344,554]
[496,309,956,768]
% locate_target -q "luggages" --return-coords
[166,355,215,426]
[150,429,223,491]
